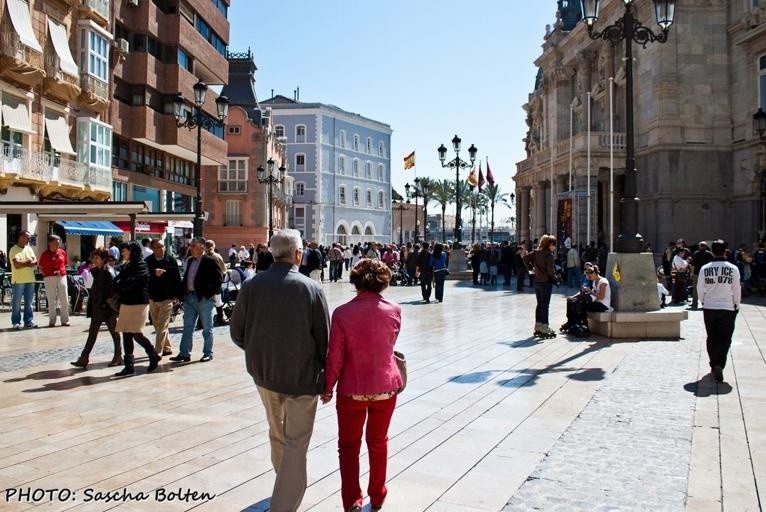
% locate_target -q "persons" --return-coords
[113,240,162,376]
[227,228,331,512]
[75,236,231,334]
[37,235,72,328]
[645,237,766,309]
[328,256,410,511]
[696,238,743,384]
[169,235,223,362]
[228,237,608,303]
[522,233,562,340]
[558,261,612,337]
[7,229,38,331]
[69,248,122,368]
[144,238,182,360]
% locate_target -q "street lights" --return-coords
[256,158,285,247]
[508,193,516,206]
[437,134,477,250]
[392,196,410,246]
[752,107,766,141]
[405,177,428,240]
[577,0,678,251]
[172,78,228,240]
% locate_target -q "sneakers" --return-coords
[25,319,38,329]
[12,323,24,330]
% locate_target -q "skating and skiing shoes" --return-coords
[533,322,557,339]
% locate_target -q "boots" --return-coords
[115,353,135,376]
[145,346,161,372]
[107,347,122,367]
[70,348,93,368]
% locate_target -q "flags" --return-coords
[402,150,415,170]
[466,166,478,192]
[477,164,485,193]
[484,160,494,188]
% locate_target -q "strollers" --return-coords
[656,265,690,307]
[213,267,247,326]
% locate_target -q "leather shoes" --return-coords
[49,324,56,327]
[61,322,70,326]
[200,354,213,362]
[169,353,191,362]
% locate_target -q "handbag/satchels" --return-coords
[393,350,407,394]
